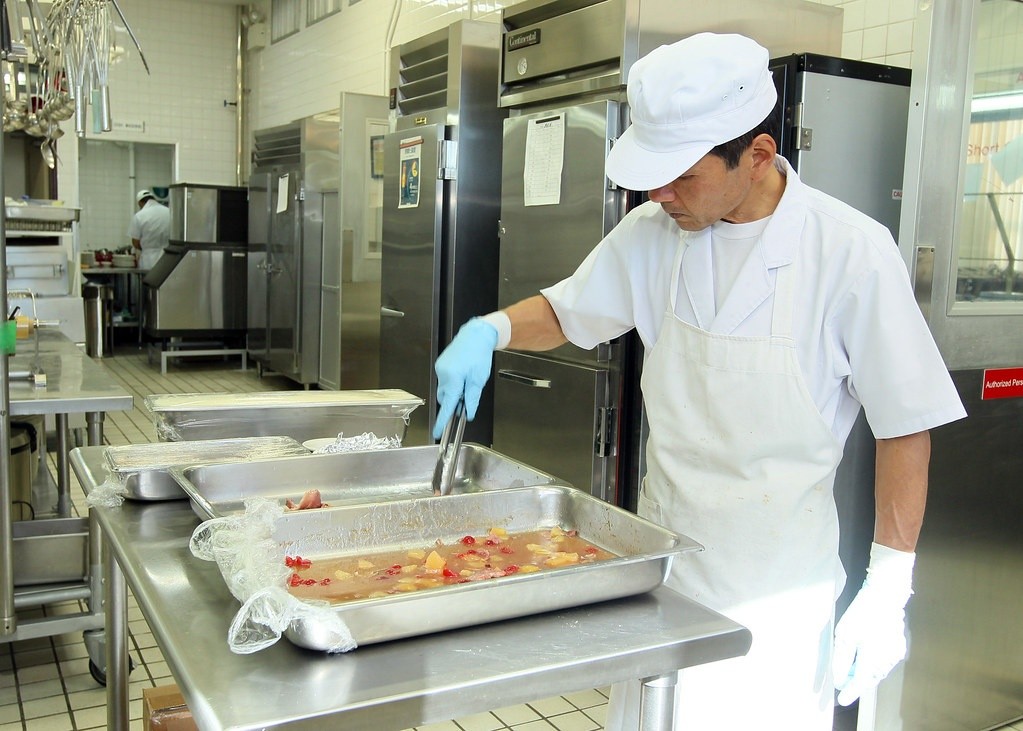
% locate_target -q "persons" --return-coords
[126,189,171,324]
[432,31,967,731]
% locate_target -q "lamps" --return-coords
[241,9,265,49]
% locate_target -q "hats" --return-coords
[605,33,778,192]
[136,190,154,210]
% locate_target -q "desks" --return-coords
[0,329,134,688]
[67,435,752,731]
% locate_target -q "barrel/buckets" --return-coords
[8,420,37,523]
[9,415,50,516]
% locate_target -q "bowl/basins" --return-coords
[101,261,112,266]
[94,262,99,266]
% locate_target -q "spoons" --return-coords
[0,58,75,169]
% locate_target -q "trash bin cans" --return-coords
[83,282,117,358]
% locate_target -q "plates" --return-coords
[112,254,136,268]
[81,252,95,268]
[303,437,348,452]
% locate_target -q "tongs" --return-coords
[432,398,469,495]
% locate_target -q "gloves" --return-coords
[433,310,511,440]
[832,542,917,707]
